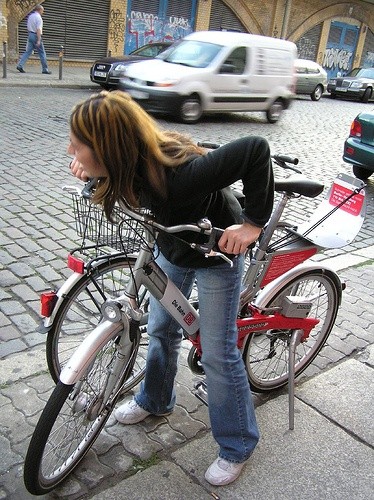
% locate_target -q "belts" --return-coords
[28,30,43,37]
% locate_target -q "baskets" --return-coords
[69,185,155,255]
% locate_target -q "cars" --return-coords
[89,41,177,94]
[293,57,329,100]
[342,109,374,180]
[327,67,374,101]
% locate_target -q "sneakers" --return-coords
[204,452,251,488]
[114,395,176,425]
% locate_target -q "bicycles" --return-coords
[39,140,306,391]
[22,179,347,497]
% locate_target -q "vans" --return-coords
[120,28,298,124]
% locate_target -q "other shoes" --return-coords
[41,70,52,75]
[16,66,26,73]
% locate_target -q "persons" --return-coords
[69,90,275,486]
[16,5,52,74]
